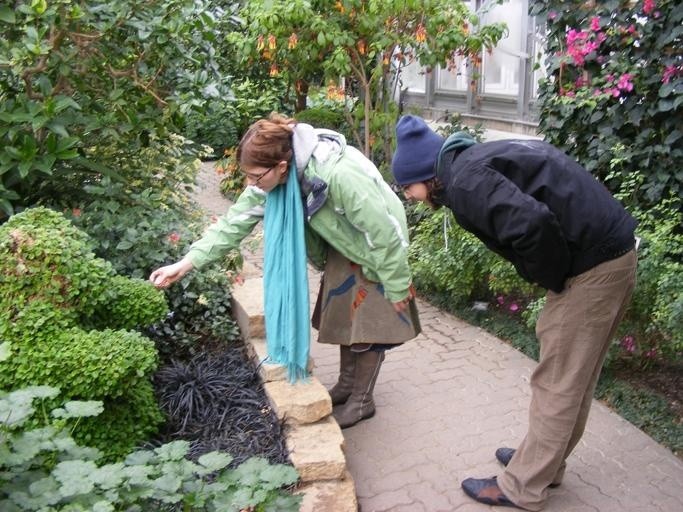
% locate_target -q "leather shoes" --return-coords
[461,476,519,507]
[495,446,562,489]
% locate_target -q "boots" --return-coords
[328,343,357,406]
[333,349,384,430]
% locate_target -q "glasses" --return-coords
[247,166,272,187]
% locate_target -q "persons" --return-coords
[390,108,641,511]
[147,110,423,433]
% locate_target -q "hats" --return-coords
[390,113,447,187]
